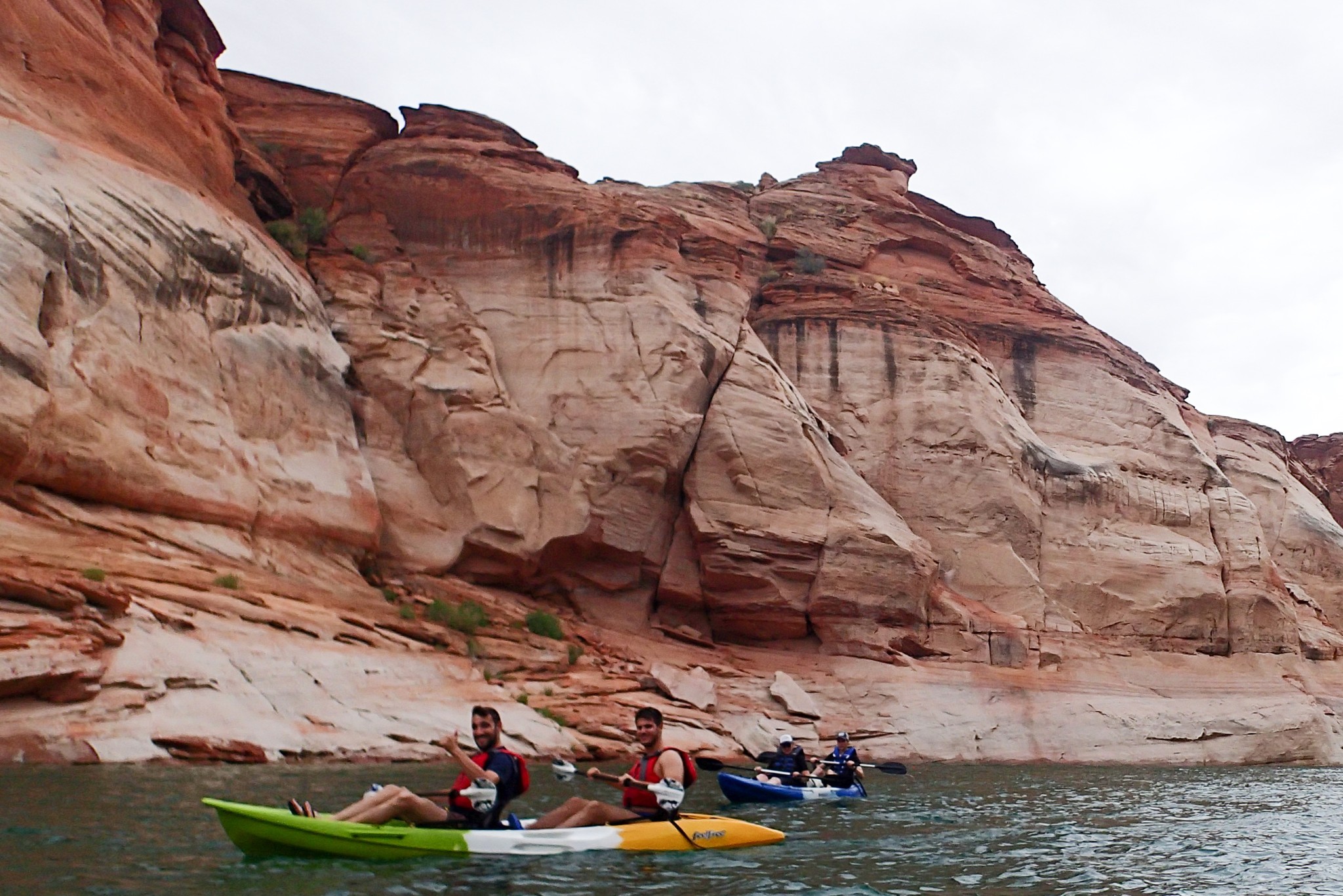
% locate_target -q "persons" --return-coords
[807,732,864,788]
[287,705,518,827]
[739,734,810,787]
[508,707,684,829]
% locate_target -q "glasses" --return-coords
[781,743,793,747]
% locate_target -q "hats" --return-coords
[779,734,793,745]
[836,732,849,741]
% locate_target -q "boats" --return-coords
[717,772,865,805]
[201,798,786,863]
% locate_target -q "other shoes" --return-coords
[288,798,305,815]
[302,801,315,818]
[507,813,525,829]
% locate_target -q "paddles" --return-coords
[695,756,854,789]
[757,751,908,775]
[363,776,498,813]
[549,758,685,810]
